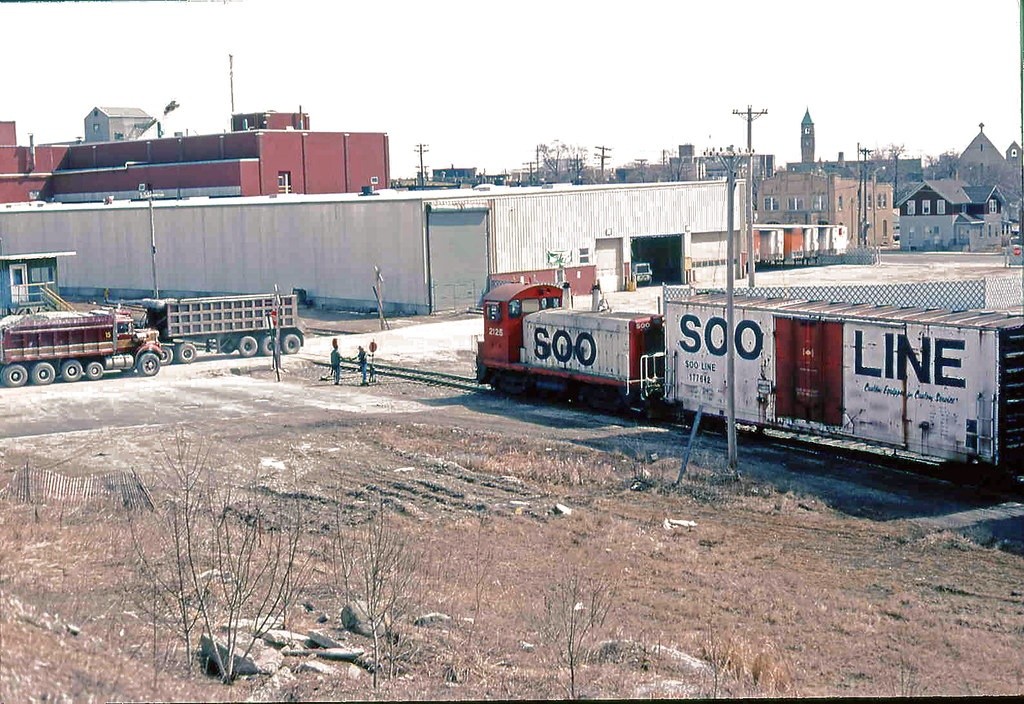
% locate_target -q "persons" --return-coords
[357,346,367,385]
[331,346,341,385]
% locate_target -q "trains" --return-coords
[476,280,1024,500]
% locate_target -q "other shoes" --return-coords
[334,382,339,385]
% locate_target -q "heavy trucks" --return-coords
[1,308,162,388]
[102,292,304,366]
